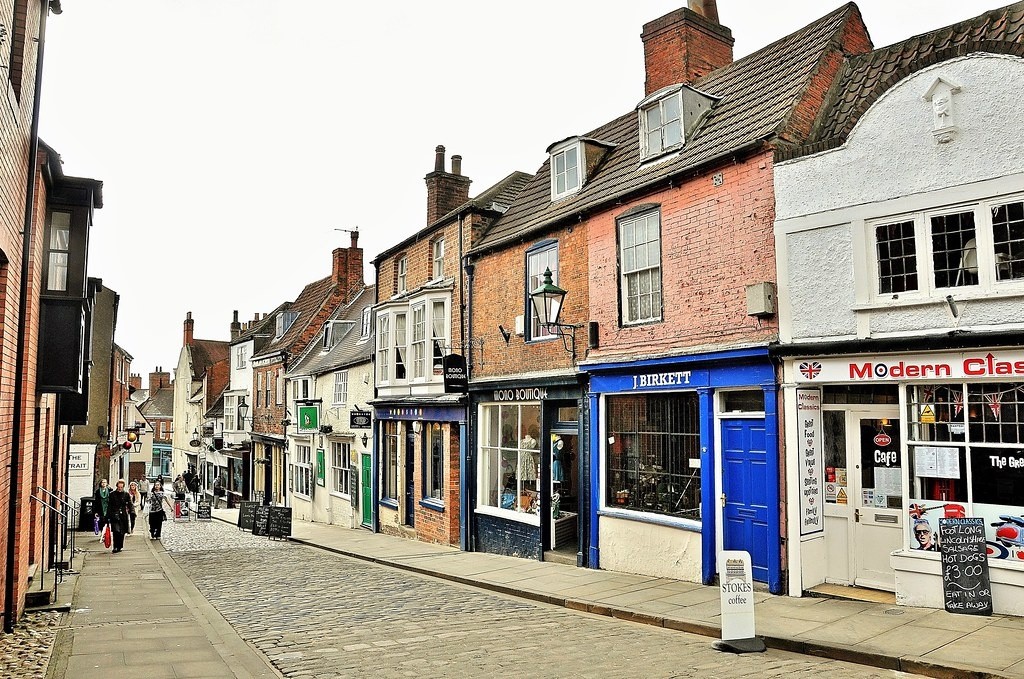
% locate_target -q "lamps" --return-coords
[529,266,583,367]
[193,428,205,447]
[237,397,256,428]
[123,426,142,454]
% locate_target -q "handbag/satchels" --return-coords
[163,511,167,521]
[105,527,111,548]
[94,519,99,535]
[99,524,111,544]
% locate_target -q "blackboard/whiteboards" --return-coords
[197,501,211,519]
[241,502,260,529]
[938,517,993,615]
[269,506,292,535]
[254,505,271,535]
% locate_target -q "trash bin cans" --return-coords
[79,497,95,531]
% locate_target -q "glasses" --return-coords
[915,529,930,535]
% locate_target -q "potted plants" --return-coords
[553,492,579,549]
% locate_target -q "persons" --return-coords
[515,434,537,480]
[126,482,141,537]
[92,478,114,541]
[146,480,173,540]
[105,479,136,553]
[139,474,150,510]
[213,476,223,509]
[156,475,164,485]
[913,518,940,552]
[173,470,201,504]
[552,434,564,482]
[133,477,140,491]
